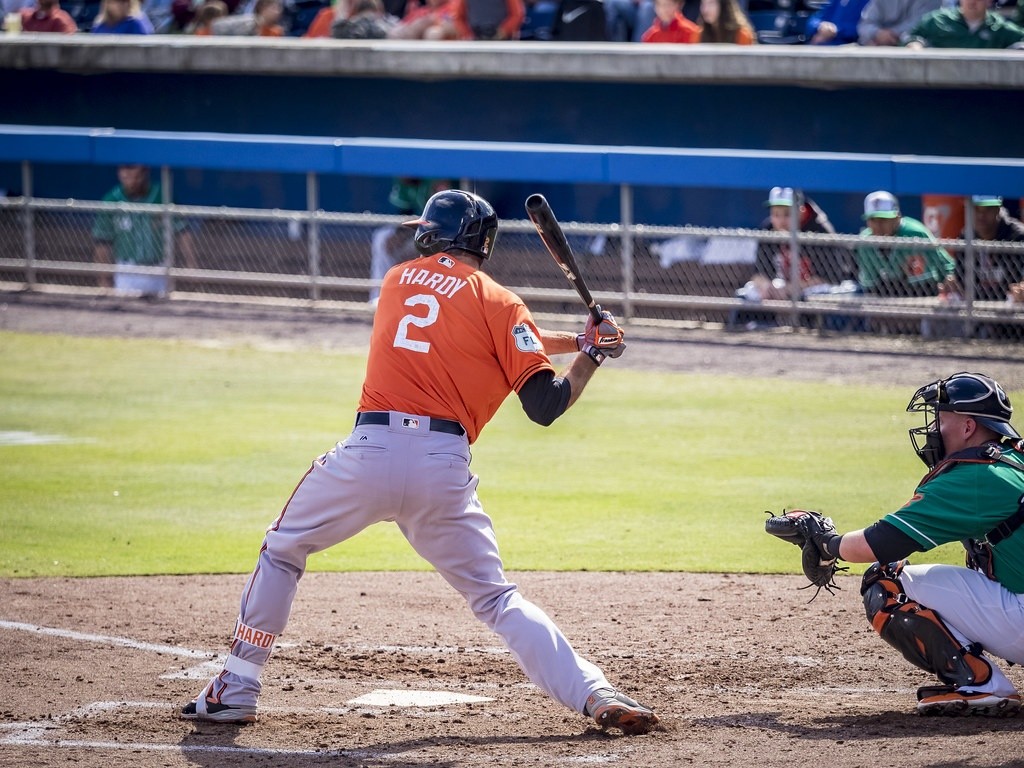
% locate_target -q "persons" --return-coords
[90,164,201,293]
[368,177,458,302]
[729,186,1024,341]
[765,372,1024,719]
[180,189,660,737]
[0,0,1024,52]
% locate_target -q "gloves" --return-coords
[576,305,627,366]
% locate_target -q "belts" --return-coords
[357,412,464,436]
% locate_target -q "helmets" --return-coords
[920,371,1023,440]
[402,190,499,258]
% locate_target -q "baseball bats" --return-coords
[523,191,602,326]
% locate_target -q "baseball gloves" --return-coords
[764,509,837,590]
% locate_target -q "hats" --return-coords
[971,194,1004,206]
[858,191,902,219]
[764,186,794,207]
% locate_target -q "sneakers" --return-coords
[917,686,1022,717]
[586,686,661,735]
[180,690,259,722]
